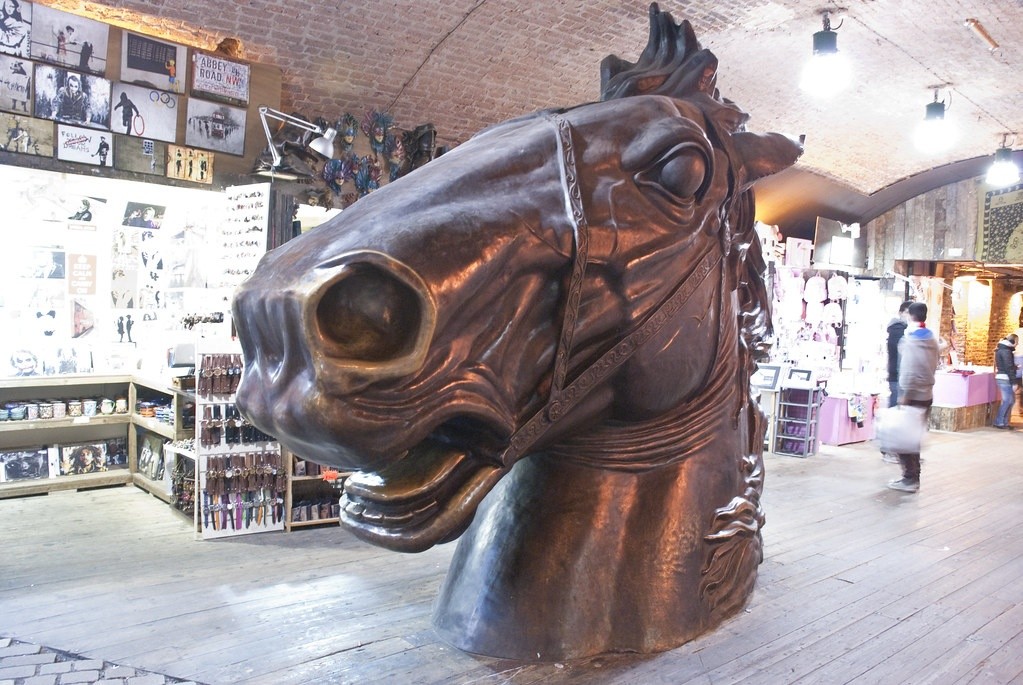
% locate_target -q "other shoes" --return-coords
[993,424,1015,430]
[889,477,921,493]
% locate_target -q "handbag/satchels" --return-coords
[874,403,927,453]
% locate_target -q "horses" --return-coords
[231,3,805,663]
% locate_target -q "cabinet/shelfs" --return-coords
[0,373,191,502]
[772,387,821,458]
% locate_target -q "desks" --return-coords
[928,367,995,431]
[822,394,877,444]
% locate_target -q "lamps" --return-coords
[258,104,338,185]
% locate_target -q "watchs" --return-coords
[199,353,286,530]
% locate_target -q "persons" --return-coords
[992,334,1019,430]
[887,302,941,492]
[49,75,92,125]
[35,247,65,278]
[72,199,92,221]
[91,136,109,166]
[880,299,925,465]
[10,350,39,376]
[56,32,67,61]
[14,128,30,153]
[0,0,26,46]
[67,446,106,476]
[123,206,160,229]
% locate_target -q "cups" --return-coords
[0,395,127,420]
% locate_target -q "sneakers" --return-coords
[882,452,901,464]
